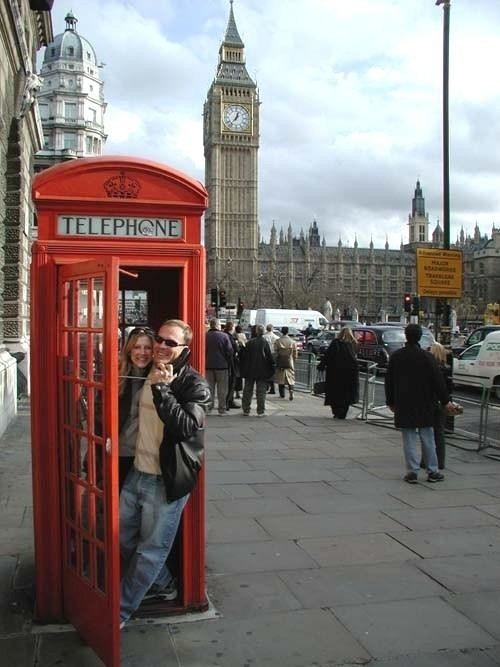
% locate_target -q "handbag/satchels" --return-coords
[313,382,328,396]
[439,400,464,417]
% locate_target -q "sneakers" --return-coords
[403,472,417,484]
[143,583,177,603]
[427,472,443,483]
[420,462,445,470]
[207,391,295,418]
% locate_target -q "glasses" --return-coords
[154,334,187,350]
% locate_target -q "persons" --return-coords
[118,319,212,631]
[420,341,451,470]
[315,327,360,419]
[384,323,458,484]
[77,315,150,405]
[304,323,318,342]
[205,315,300,415]
[87,324,156,508]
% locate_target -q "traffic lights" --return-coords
[238,302,244,319]
[211,287,226,307]
[405,293,419,316]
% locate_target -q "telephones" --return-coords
[164,348,190,376]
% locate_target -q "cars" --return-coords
[217,303,500,400]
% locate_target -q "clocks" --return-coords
[222,102,251,132]
